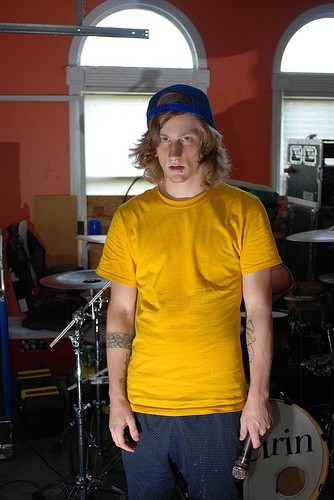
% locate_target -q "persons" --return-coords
[96,83,284,500]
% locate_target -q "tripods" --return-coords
[31,280,124,500]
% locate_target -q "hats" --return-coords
[146,84,217,129]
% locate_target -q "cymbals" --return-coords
[239,311,288,318]
[318,272,334,284]
[286,230,333,243]
[39,268,112,288]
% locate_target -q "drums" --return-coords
[242,398,330,500]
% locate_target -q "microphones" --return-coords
[232,433,252,480]
